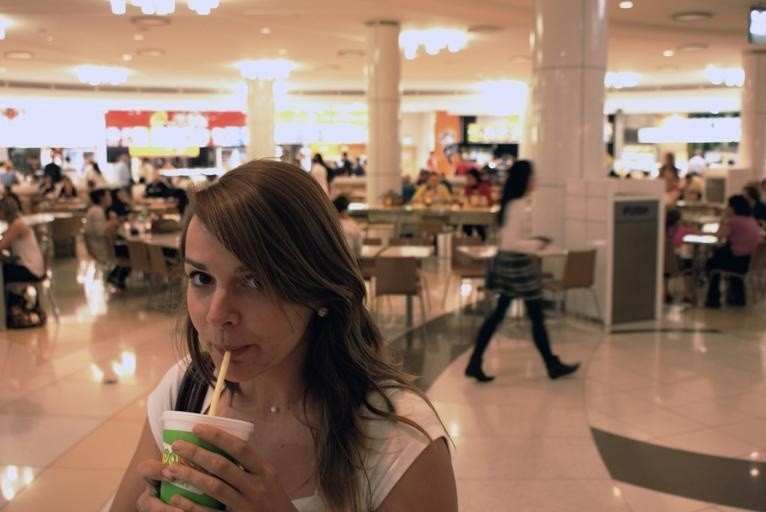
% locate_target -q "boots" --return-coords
[466,358,494,381]
[544,355,580,379]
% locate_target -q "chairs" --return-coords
[0,169,603,350]
[667,199,765,312]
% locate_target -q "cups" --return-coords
[157,409,255,509]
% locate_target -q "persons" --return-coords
[1,147,195,329]
[657,149,766,307]
[311,151,366,196]
[403,166,494,242]
[461,157,584,386]
[332,196,363,256]
[101,156,461,512]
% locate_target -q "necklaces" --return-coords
[262,387,302,414]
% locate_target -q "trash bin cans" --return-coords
[50,212,76,259]
